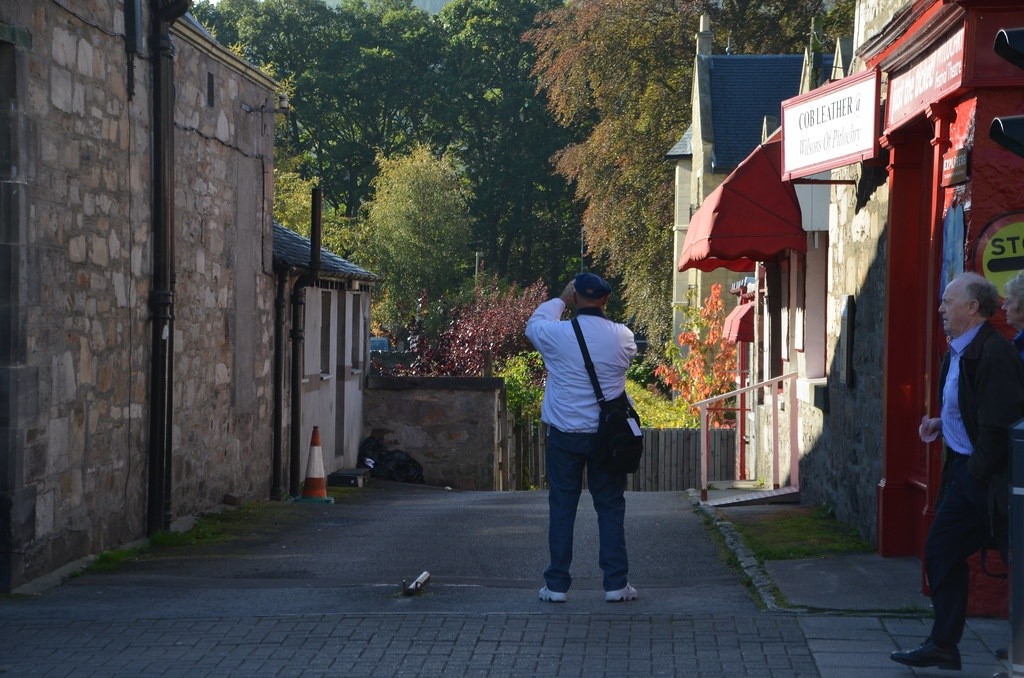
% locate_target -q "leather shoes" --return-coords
[890,636,961,671]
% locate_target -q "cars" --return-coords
[369,337,392,354]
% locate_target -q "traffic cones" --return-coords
[295,426,334,505]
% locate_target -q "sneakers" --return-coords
[539,580,567,602]
[605,582,637,601]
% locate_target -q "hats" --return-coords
[574,271,612,299]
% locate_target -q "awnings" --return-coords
[722,303,754,343]
[677,139,807,272]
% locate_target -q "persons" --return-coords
[889,272,1024,669]
[525,272,638,603]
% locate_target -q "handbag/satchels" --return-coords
[597,390,643,474]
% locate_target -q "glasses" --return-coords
[1001,297,1018,307]
